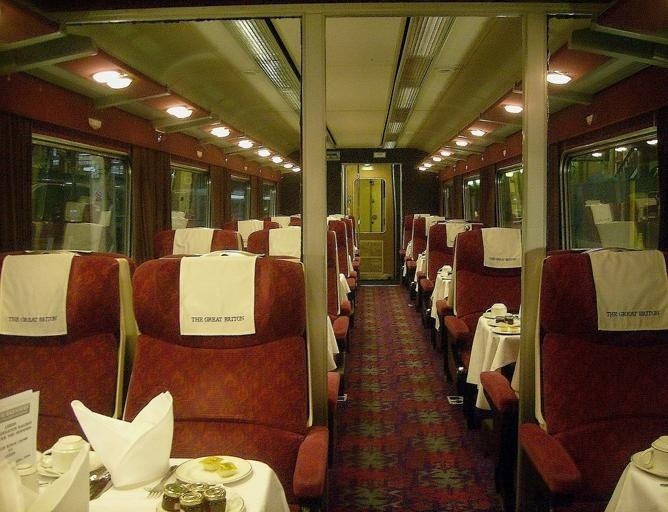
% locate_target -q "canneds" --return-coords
[161,481,226,512]
[496,315,514,325]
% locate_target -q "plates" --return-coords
[484,304,520,335]
[155,456,253,512]
[631,451,668,478]
[38,450,102,477]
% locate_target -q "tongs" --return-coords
[90,464,111,500]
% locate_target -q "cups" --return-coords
[40,436,90,472]
[640,436,668,474]
[422,252,426,256]
[438,265,452,280]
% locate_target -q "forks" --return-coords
[145,464,177,499]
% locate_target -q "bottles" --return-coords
[16,465,39,494]
